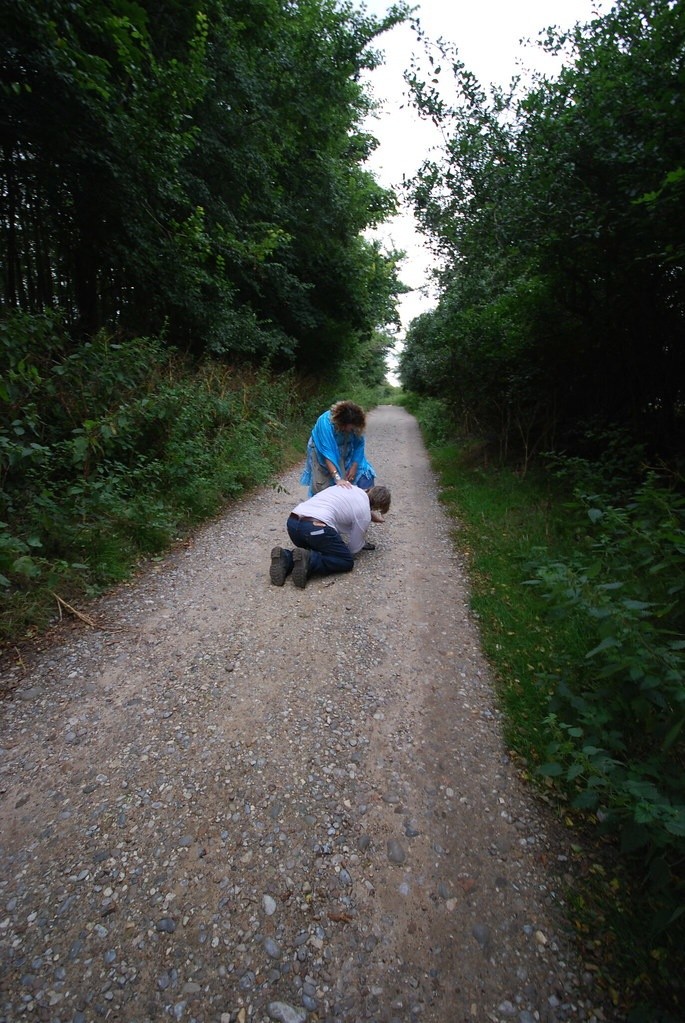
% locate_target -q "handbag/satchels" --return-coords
[312,454,348,494]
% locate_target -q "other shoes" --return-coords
[292,548,309,588]
[371,511,385,522]
[270,546,287,586]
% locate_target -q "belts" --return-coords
[289,512,323,523]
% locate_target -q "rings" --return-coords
[351,478,355,480]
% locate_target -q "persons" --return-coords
[268,480,390,588]
[301,400,385,524]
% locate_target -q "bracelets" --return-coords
[332,475,340,481]
[330,471,338,475]
